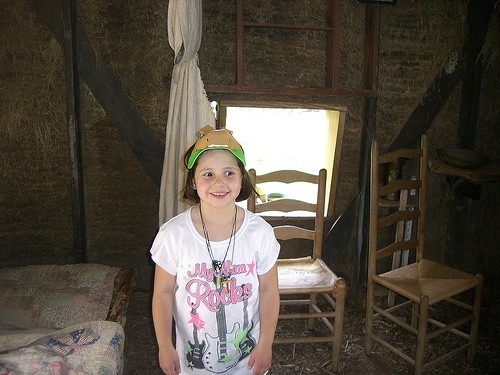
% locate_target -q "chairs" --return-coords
[364,133,484,375]
[248,166,347,375]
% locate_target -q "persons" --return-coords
[149,126,282,375]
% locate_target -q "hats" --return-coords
[187,125,246,170]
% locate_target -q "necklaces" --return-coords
[198,201,238,289]
